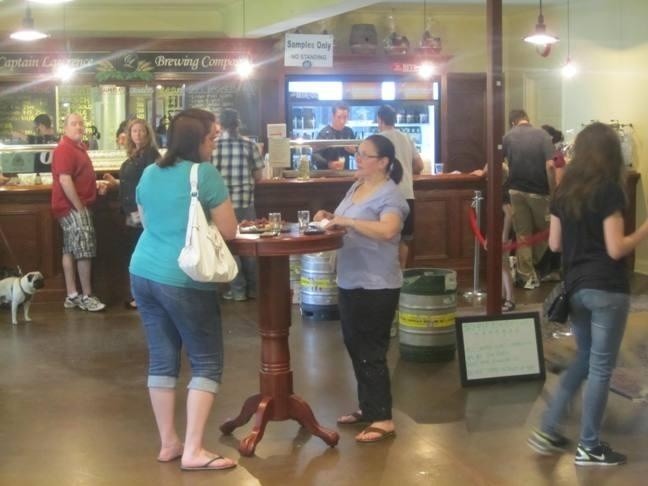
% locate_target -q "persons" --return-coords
[310,103,358,170]
[127,108,238,471]
[8,112,162,313]
[209,108,266,302]
[312,134,411,443]
[375,104,424,269]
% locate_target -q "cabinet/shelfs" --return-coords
[292,122,431,169]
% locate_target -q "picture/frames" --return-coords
[453,312,547,388]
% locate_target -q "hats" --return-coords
[219,108,240,119]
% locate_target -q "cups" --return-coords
[435,163,444,175]
[297,210,310,233]
[268,212,281,237]
[296,154,312,180]
[273,167,283,178]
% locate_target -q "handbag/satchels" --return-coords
[127,212,142,229]
[177,195,240,283]
[542,281,568,324]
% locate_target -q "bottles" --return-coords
[393,103,429,124]
[290,105,316,130]
[292,128,421,145]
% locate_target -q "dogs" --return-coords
[0,270,46,324]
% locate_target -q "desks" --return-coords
[219,220,346,456]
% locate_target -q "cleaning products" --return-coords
[297,147,310,180]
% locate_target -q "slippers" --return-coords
[356,426,396,444]
[336,410,369,424]
[178,454,236,471]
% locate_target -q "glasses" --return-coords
[355,151,381,160]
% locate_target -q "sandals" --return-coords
[502,300,515,312]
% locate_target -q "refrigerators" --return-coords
[287,73,440,175]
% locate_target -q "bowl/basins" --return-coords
[38,172,53,185]
[16,173,37,186]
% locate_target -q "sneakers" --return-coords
[526,428,571,456]
[78,294,107,312]
[574,439,629,468]
[221,290,247,301]
[63,294,82,309]
[524,277,540,290]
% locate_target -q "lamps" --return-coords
[9,1,51,42]
[415,0,435,81]
[519,0,560,45]
[235,0,254,80]
[55,1,72,83]
[562,0,577,80]
